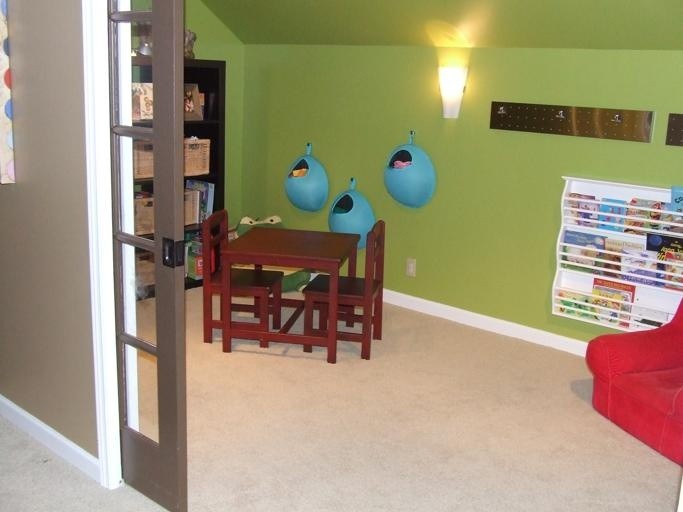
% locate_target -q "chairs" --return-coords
[302,220,385,360]
[585,299,683,466]
[202,210,284,348]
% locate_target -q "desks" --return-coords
[220,228,361,363]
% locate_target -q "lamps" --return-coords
[438,61,467,118]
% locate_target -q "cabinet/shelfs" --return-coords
[131,54,225,288]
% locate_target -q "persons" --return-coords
[132,82,152,121]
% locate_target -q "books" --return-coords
[186,180,215,223]
[558,181,683,330]
